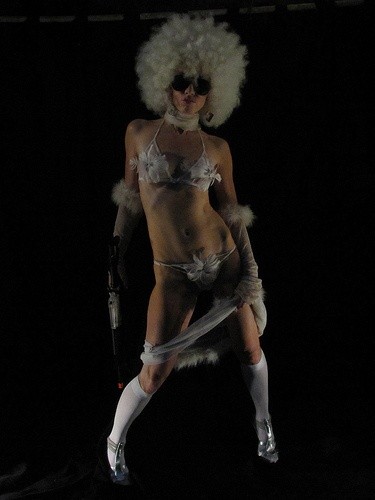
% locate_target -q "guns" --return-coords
[106,265,122,386]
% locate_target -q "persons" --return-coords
[103,14,279,484]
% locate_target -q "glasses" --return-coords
[171,75,211,95]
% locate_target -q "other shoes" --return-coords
[256,415,279,464]
[107,437,129,485]
